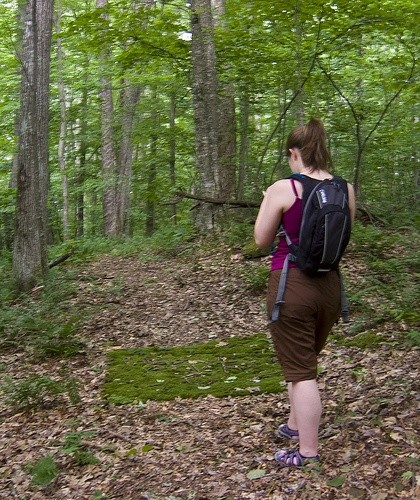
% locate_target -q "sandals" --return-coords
[274,449,321,470]
[278,423,299,441]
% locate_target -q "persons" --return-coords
[251,116,358,468]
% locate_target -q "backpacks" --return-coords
[279,173,353,279]
